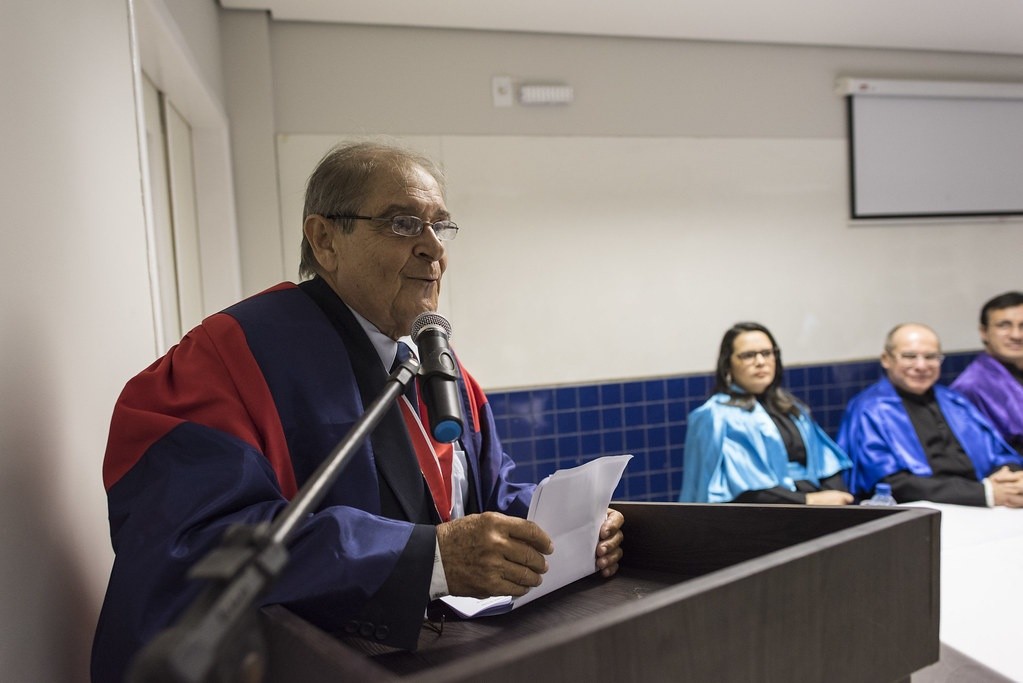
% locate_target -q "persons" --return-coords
[90,142,623,683]
[676,323,855,506]
[947,292,1023,457]
[837,323,1023,509]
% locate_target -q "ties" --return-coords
[388,342,421,422]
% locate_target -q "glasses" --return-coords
[324,215,459,241]
[735,347,775,365]
[897,349,946,368]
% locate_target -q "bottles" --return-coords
[867,484,898,507]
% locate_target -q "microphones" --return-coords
[411,311,464,444]
